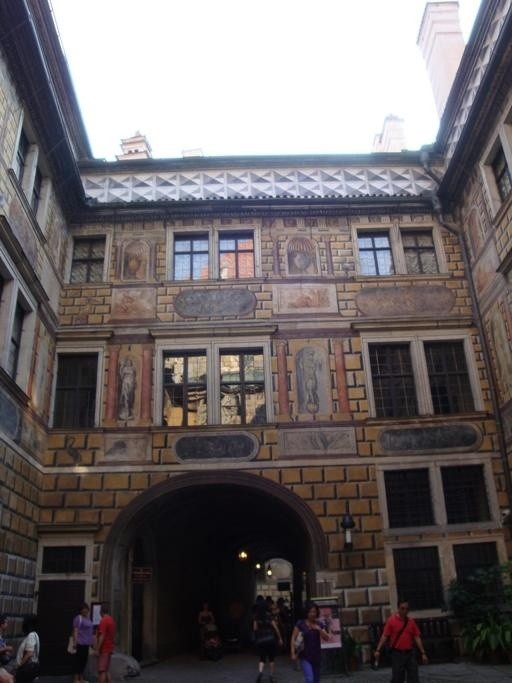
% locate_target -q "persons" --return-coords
[71,601,116,683]
[290,602,331,683]
[240,595,289,683]
[0,614,42,683]
[197,600,224,661]
[373,598,429,683]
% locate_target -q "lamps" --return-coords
[340,515,357,552]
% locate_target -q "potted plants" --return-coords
[445,552,511,664]
[324,628,363,676]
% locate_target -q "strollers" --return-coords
[204,624,225,661]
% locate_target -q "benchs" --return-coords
[369,615,460,669]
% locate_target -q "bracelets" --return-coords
[375,650,381,652]
[420,652,427,656]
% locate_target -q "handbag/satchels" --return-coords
[295,632,304,651]
[255,633,275,649]
[370,647,391,669]
[16,662,40,682]
[67,636,77,654]
